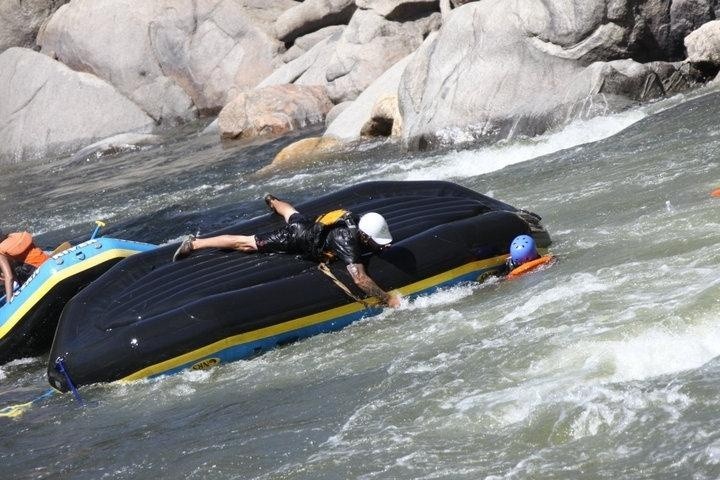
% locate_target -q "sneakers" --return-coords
[172,233,197,266]
[261,191,282,212]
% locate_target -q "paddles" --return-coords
[0,242,71,302]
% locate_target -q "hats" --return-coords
[357,211,395,248]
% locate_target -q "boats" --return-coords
[39,176,558,401]
[0,236,169,369]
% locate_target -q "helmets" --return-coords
[508,233,538,267]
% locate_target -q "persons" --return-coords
[0,228,51,303]
[477,234,541,286]
[170,193,406,309]
